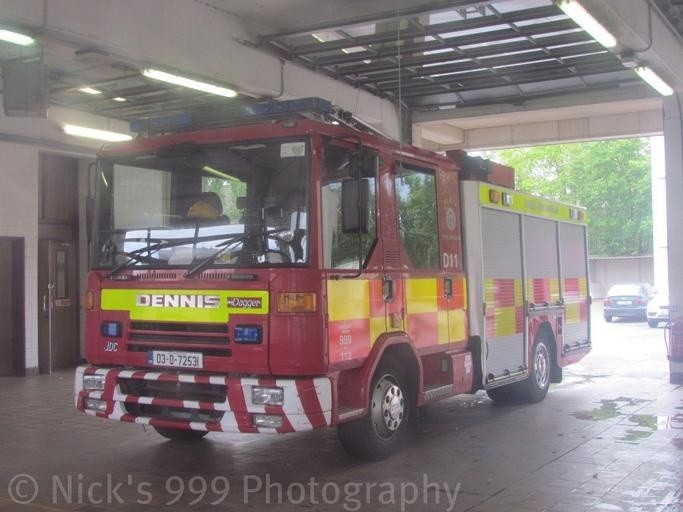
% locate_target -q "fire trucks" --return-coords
[74,96,592,464]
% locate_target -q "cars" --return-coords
[648,288,669,328]
[603,282,652,322]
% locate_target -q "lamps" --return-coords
[63,124,133,142]
[633,60,674,96]
[139,67,237,99]
[555,0,617,48]
[0,30,34,48]
